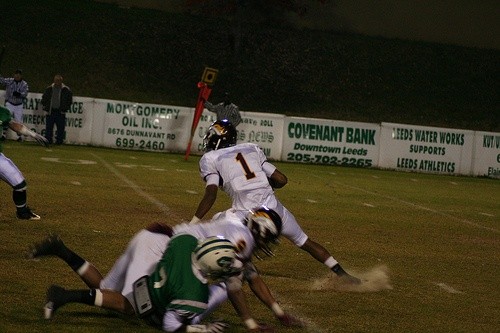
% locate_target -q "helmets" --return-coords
[189,236,246,286]
[241,206,284,247]
[198,119,239,152]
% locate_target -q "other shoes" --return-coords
[16,211,41,220]
[43,283,72,320]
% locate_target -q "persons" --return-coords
[0,69,30,141]
[204,91,241,127]
[188,119,362,326]
[32,206,306,333]
[132,234,243,333]
[0,106,49,221]
[41,72,72,144]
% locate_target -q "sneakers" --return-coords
[33,235,64,261]
